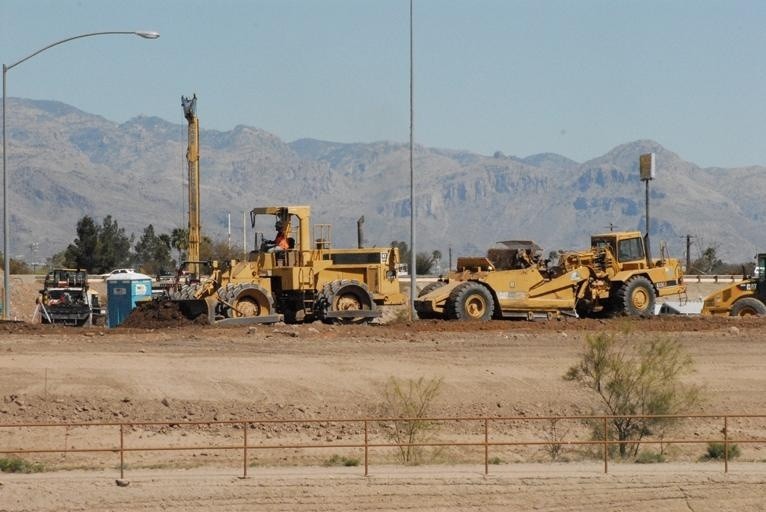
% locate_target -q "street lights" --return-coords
[3,32,160,319]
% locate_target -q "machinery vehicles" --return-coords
[700,252,766,319]
[38,262,100,328]
[412,229,688,320]
[163,208,406,326]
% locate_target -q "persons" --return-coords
[609,241,616,257]
[512,248,534,270]
[617,241,629,259]
[263,222,290,253]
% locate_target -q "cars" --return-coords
[98,265,154,283]
